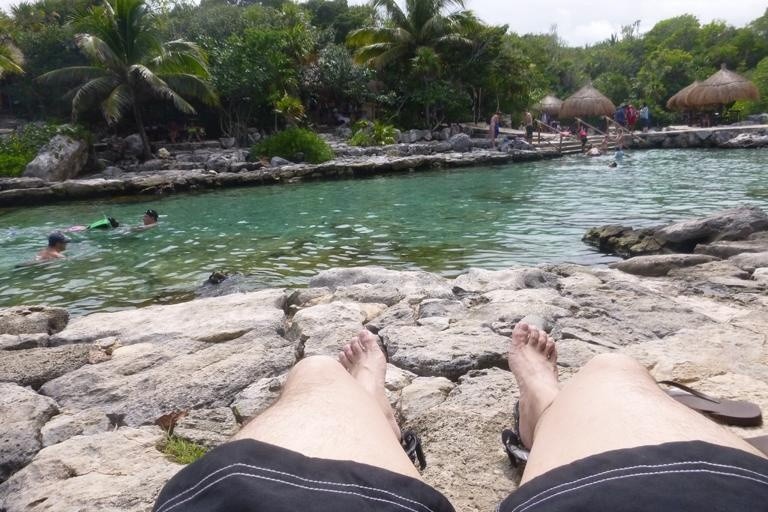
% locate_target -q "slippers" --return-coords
[657,381,761,425]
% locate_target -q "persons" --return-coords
[153,321,767,512]
[128,209,159,233]
[626,105,640,134]
[614,105,629,135]
[576,128,635,171]
[490,111,502,151]
[541,112,550,131]
[639,102,648,133]
[702,114,711,127]
[33,233,72,262]
[69,217,119,232]
[521,109,533,145]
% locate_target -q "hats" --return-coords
[48,233,70,244]
